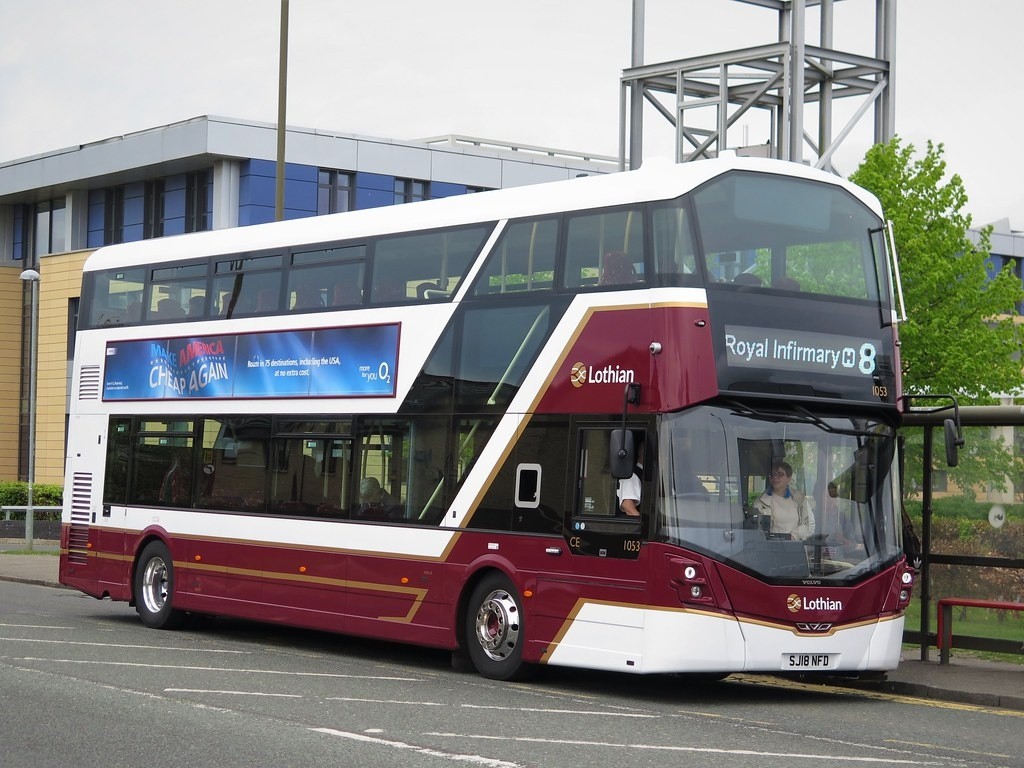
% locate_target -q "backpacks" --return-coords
[903,524,923,575]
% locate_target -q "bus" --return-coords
[58,156,966,688]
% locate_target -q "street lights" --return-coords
[17,268,43,548]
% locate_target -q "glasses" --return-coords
[769,471,787,479]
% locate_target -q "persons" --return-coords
[752,461,816,541]
[616,440,644,516]
[826,497,855,547]
[360,476,402,518]
